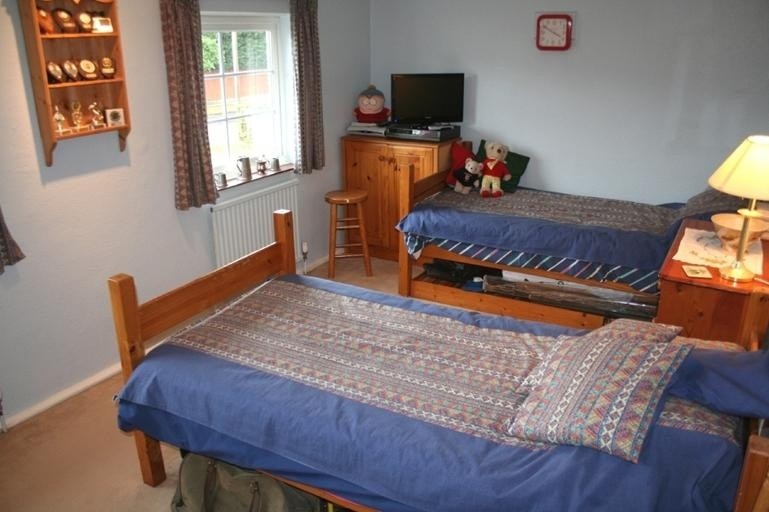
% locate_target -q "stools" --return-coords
[325,187,374,280]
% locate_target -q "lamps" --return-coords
[709,134,769,283]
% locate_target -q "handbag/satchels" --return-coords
[170,453,329,511]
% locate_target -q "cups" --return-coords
[270,158,279,170]
[256,159,267,176]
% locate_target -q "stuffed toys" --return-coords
[477,140,512,197]
[453,158,483,194]
[353,84,391,127]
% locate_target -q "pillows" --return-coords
[473,140,531,192]
[517,316,685,393]
[662,188,749,243]
[506,333,697,462]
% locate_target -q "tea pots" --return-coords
[235,155,252,181]
[212,172,227,188]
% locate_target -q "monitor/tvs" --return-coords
[390,73,464,124]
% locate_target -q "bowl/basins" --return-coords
[710,213,769,251]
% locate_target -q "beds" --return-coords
[399,139,753,330]
[108,208,769,512]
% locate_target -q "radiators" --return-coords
[210,178,309,276]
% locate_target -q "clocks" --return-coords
[536,14,572,49]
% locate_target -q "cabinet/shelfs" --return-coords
[341,134,473,268]
[17,0,131,172]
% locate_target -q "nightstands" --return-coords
[655,217,769,352]
